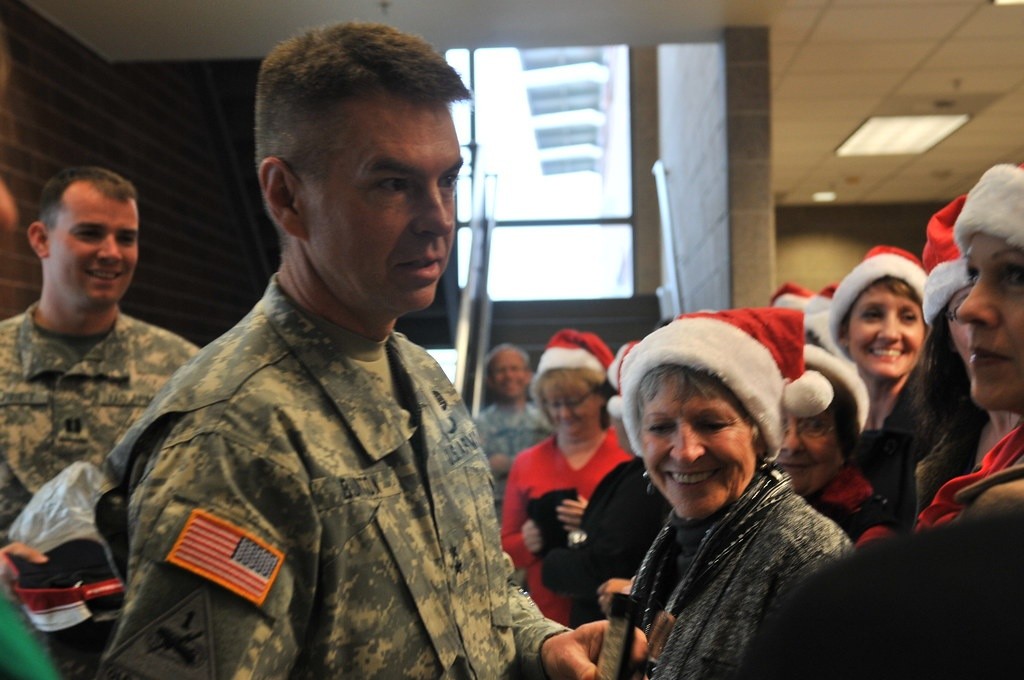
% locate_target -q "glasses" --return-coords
[543,389,594,410]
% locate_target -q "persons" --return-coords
[90,19,649,680]
[468,343,561,523]
[912,161,1024,529]
[913,194,1024,515]
[778,340,899,552]
[0,176,64,678]
[826,246,936,527]
[1,162,202,679]
[501,326,631,628]
[620,301,857,680]
[539,337,675,631]
[732,513,1024,679]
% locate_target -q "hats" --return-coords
[609,341,642,395]
[527,326,622,418]
[829,247,928,362]
[802,340,871,436]
[767,281,840,358]
[620,306,835,458]
[922,190,972,327]
[954,159,1024,269]
